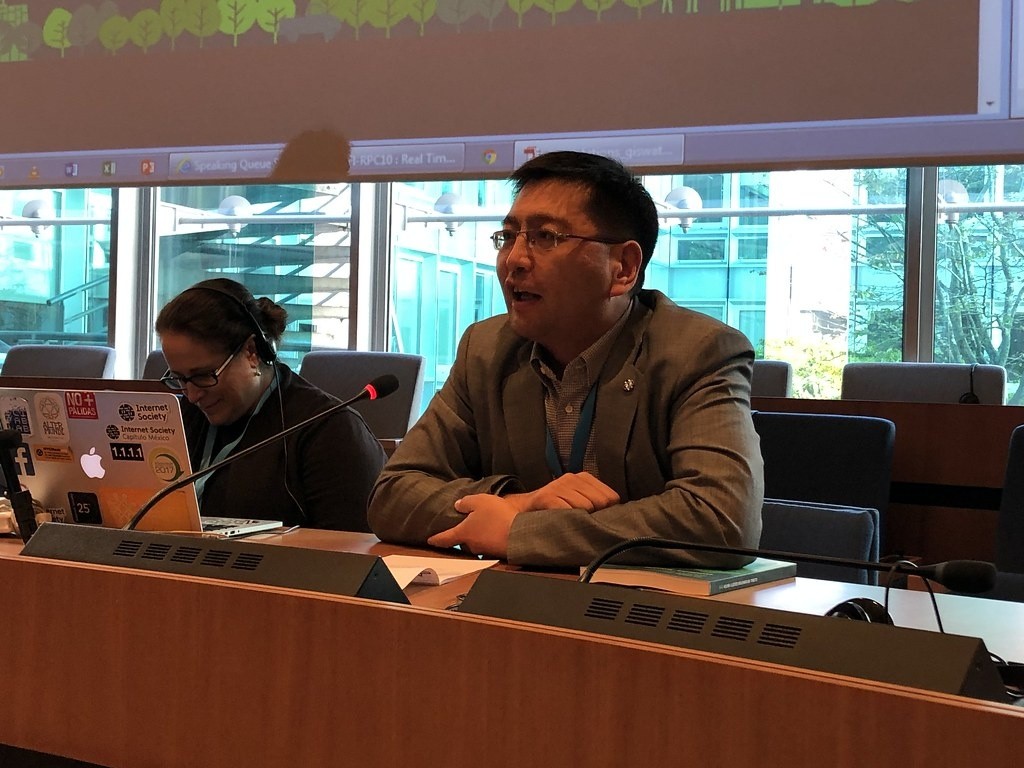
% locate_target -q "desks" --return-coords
[748,399,1024,559]
[1,517,1024,767]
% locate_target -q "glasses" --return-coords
[160,340,246,390]
[490,229,624,250]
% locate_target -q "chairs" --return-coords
[947,425,1024,597]
[0,345,117,381]
[298,351,427,436]
[751,411,896,583]
[840,362,1007,406]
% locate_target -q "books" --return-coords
[579,558,797,597]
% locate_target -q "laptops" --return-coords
[0,388,283,538]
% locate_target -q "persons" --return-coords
[365,150,766,574]
[154,278,389,535]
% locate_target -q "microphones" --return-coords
[120,375,400,532]
[576,537,999,598]
